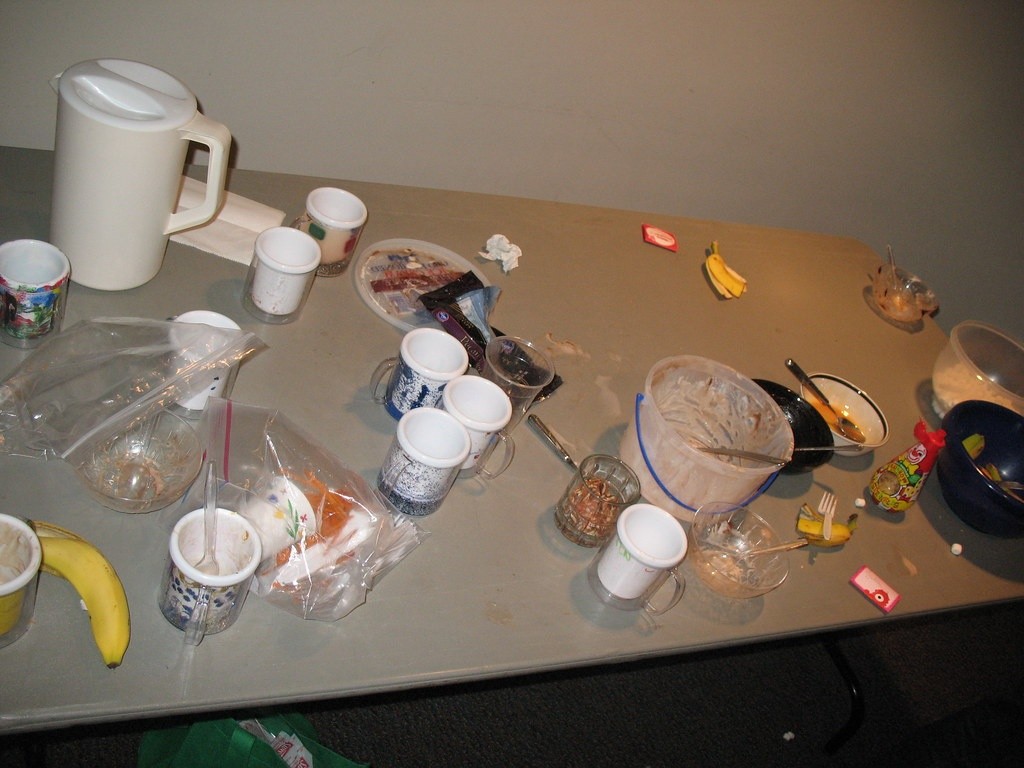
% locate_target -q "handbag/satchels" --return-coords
[135,704,370,768]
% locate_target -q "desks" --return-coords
[0,146,1024,768]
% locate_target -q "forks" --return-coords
[818,491,838,540]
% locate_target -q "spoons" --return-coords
[193,461,219,578]
[116,411,159,499]
[785,358,866,443]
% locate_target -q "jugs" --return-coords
[47,56,232,291]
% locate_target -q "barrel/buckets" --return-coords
[620,355,795,521]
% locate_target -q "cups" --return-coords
[589,503,688,615]
[368,327,469,421]
[482,334,555,435]
[236,476,317,564]
[167,310,245,420]
[242,227,321,324]
[286,187,368,277]
[0,512,43,649]
[438,374,515,481]
[156,503,262,647]
[0,239,70,350]
[377,407,471,519]
[554,454,641,549]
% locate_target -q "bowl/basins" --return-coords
[873,265,938,322]
[800,372,890,457]
[66,403,204,515]
[931,319,1024,420]
[938,399,1023,538]
[750,379,836,475]
[687,500,790,600]
